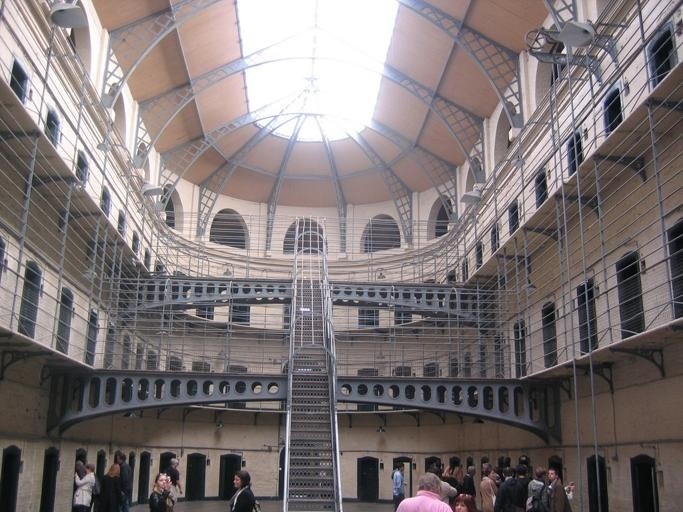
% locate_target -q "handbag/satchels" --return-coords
[526,496,540,512]
[489,479,497,508]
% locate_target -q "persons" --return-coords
[149,473,177,512]
[229,470,256,512]
[391,462,576,512]
[165,457,184,494]
[72,452,133,512]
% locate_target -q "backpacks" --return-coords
[252,500,261,512]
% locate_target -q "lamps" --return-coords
[377,426,385,433]
[460,188,482,204]
[521,280,537,291]
[556,20,595,50]
[472,417,484,424]
[222,262,235,279]
[96,138,112,151]
[123,411,138,419]
[81,266,97,278]
[131,174,164,196]
[375,267,386,282]
[51,3,86,31]
[216,420,224,429]
